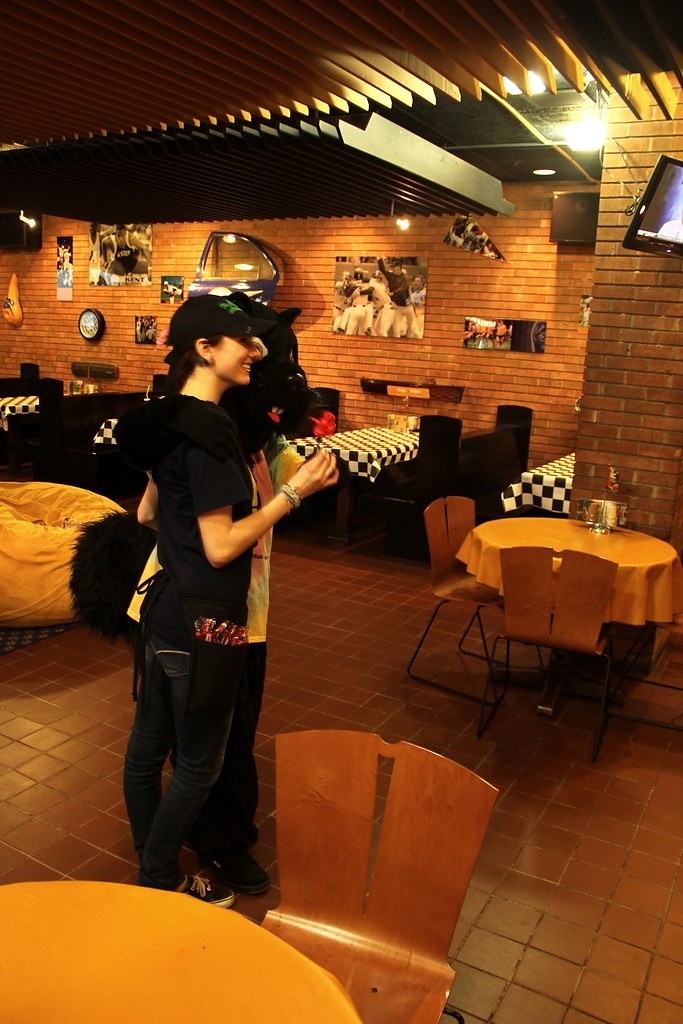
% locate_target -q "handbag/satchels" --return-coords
[69,510,160,646]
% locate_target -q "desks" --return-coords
[0,881,364,1024]
[286,427,419,549]
[501,451,575,513]
[455,517,683,707]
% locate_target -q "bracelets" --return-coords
[148,267,152,270]
[279,483,301,514]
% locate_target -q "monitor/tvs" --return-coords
[622,153,683,258]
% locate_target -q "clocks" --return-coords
[78,308,106,342]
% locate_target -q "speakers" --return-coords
[549,190,600,246]
[0,210,43,249]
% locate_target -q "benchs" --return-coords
[0,362,532,563]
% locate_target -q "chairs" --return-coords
[476,545,637,767]
[401,494,546,707]
[257,729,501,1024]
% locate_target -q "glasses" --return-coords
[164,285,166,286]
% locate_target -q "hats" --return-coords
[169,294,278,344]
[355,268,369,274]
[336,281,344,288]
[164,281,168,285]
[226,292,302,324]
[388,261,402,267]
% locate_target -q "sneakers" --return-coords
[197,842,269,894]
[175,874,235,908]
[181,836,199,854]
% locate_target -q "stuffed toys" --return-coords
[69,290,350,894]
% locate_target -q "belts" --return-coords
[353,305,365,307]
[333,305,344,312]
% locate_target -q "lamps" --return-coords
[19,210,36,228]
[388,200,410,231]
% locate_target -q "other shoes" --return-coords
[365,331,370,336]
[337,328,344,332]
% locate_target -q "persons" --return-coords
[163,281,182,303]
[446,215,492,255]
[466,320,512,346]
[122,294,340,908]
[333,257,426,339]
[89,221,152,287]
[135,316,157,341]
[57,244,72,287]
